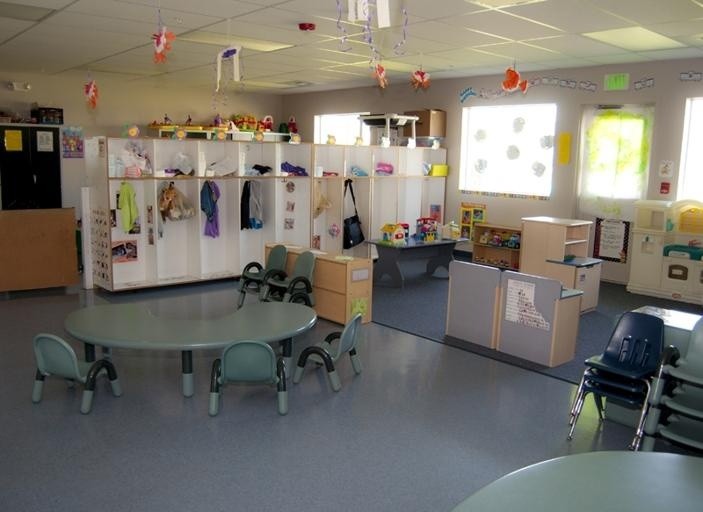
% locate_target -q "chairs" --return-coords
[235,244,316,308]
[209,340,288,416]
[32,334,123,414]
[567,312,703,451]
[293,313,361,392]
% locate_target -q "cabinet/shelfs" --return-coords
[445,215,604,369]
[265,242,373,326]
[625,224,702,304]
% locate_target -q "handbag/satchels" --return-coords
[344,215,364,249]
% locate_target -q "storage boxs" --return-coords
[30,107,63,124]
[404,107,447,136]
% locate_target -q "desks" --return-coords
[450,452,703,511]
[0,207,78,300]
[357,110,418,147]
[363,236,469,288]
[65,288,317,397]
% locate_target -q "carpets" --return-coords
[232,248,703,385]
